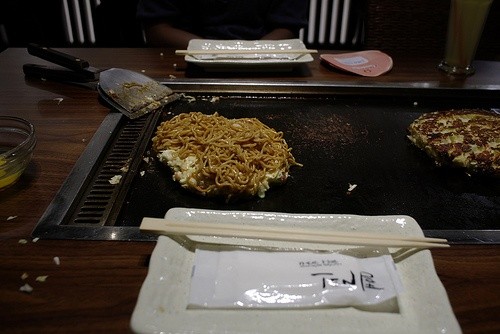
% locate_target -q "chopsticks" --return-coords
[176,49,319,55]
[140,217,451,248]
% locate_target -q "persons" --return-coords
[135,0,310,48]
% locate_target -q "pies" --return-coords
[411,107,500,171]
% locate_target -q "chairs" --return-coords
[61,0,368,51]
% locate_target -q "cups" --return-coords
[438,0,493,76]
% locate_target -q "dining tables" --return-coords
[0,48,500,334]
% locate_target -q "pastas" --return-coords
[154,110,303,195]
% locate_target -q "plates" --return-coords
[185,38,314,72]
[128,207,462,334]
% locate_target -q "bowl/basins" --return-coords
[0,115,39,192]
